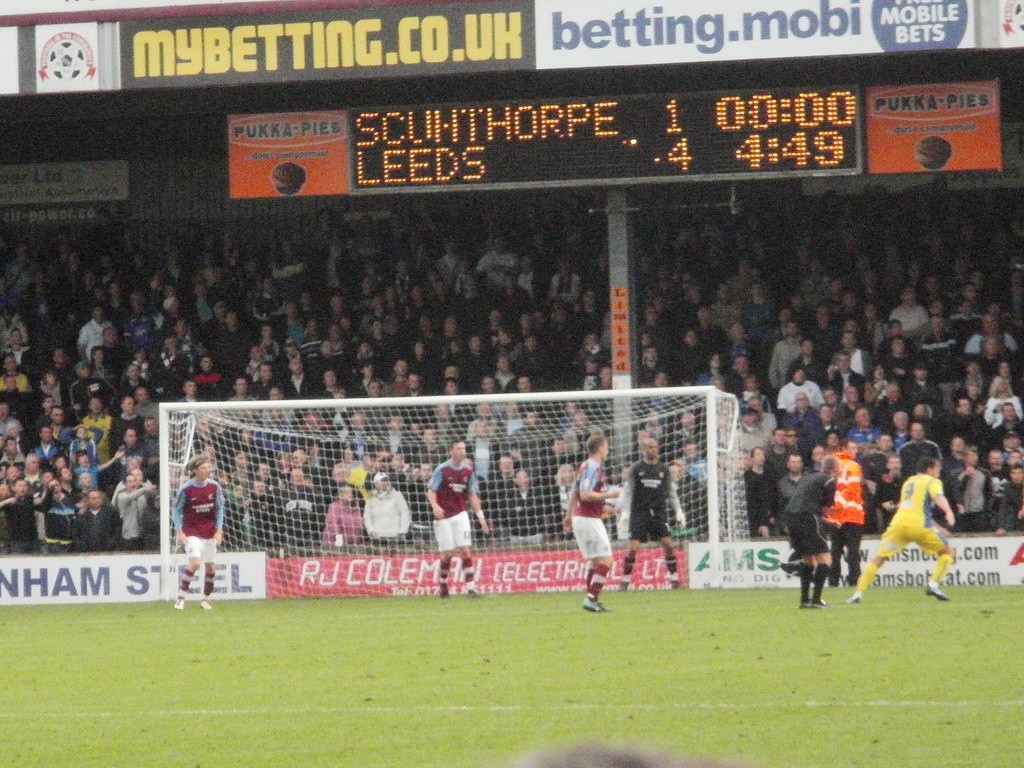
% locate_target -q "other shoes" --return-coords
[807,599,827,608]
[200,601,212,610]
[582,597,606,611]
[925,582,949,601]
[799,598,813,607]
[845,592,862,603]
[174,597,185,610]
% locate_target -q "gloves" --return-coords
[335,534,343,547]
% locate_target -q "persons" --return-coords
[847,457,955,603]
[618,437,686,591]
[171,454,223,610]
[562,435,620,612]
[0,183,1024,554]
[427,440,490,599]
[784,439,866,609]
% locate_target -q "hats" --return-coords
[189,454,209,469]
[374,472,388,483]
[75,360,87,369]
